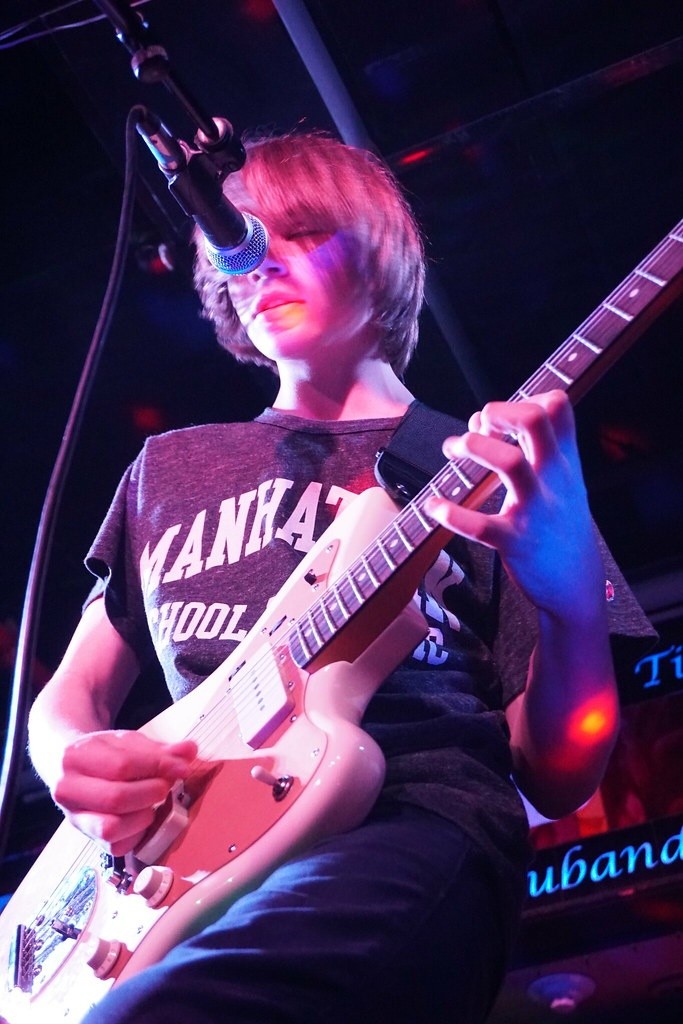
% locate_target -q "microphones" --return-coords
[131,105,271,276]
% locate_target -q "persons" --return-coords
[28,132,617,1023]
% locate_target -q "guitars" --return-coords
[0,217,683,1024]
